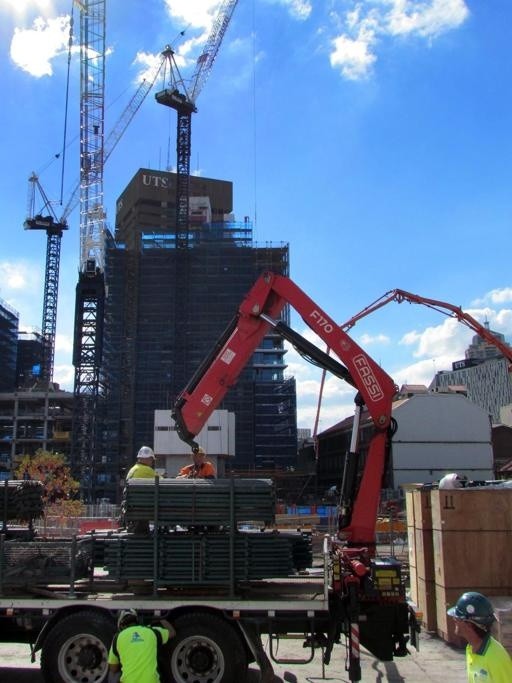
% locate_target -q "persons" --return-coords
[106,608,177,683]
[176,446,216,480]
[125,445,164,479]
[445,591,512,683]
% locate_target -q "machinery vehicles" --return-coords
[312,288,512,470]
[0,270,420,683]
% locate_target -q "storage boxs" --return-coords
[406,488,512,646]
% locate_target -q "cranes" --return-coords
[22,44,174,396]
[68,0,113,505]
[154,0,241,407]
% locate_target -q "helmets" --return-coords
[447,591,495,624]
[137,445,156,460]
[197,445,206,455]
